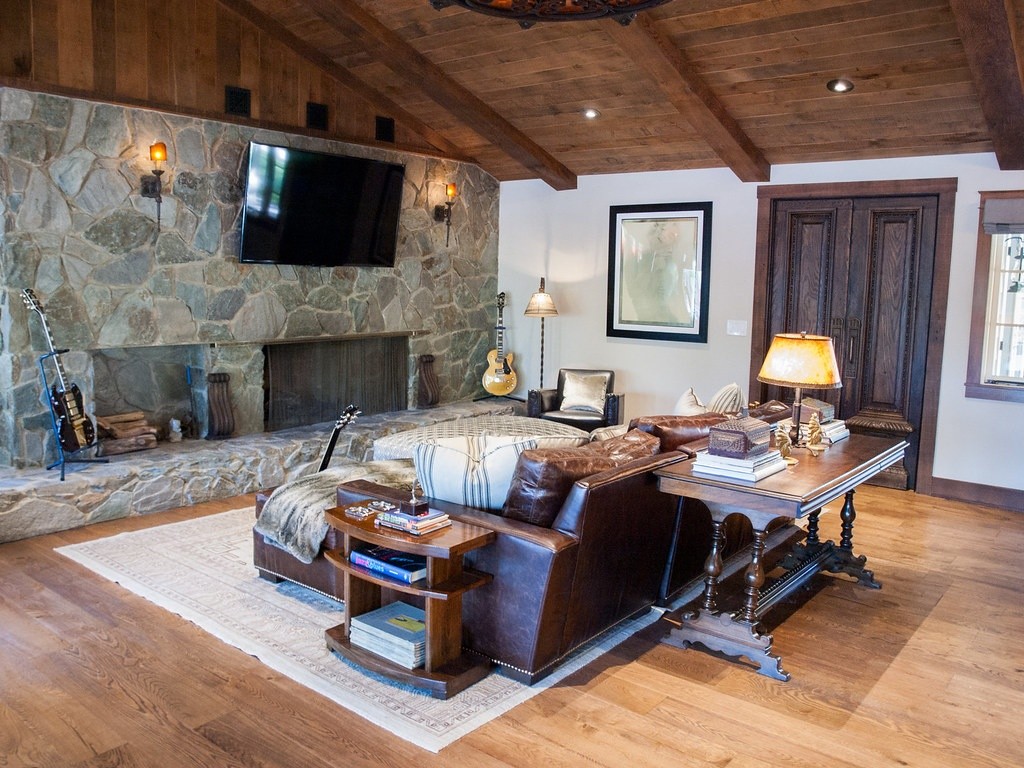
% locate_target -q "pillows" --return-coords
[413,434,589,510]
[590,424,629,442]
[674,387,708,416]
[708,383,746,414]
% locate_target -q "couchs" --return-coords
[254,401,795,687]
[560,372,608,415]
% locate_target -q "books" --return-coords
[690,448,789,482]
[349,508,452,670]
[777,417,850,444]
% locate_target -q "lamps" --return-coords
[140,142,168,232]
[434,182,457,247]
[523,278,559,389]
[757,331,843,448]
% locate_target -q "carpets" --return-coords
[52,504,831,754]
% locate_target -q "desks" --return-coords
[652,433,911,682]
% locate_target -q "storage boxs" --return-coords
[709,416,770,459]
[800,397,834,424]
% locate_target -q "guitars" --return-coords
[18,287,94,453]
[481,291,518,396]
[315,403,363,472]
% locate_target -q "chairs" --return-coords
[527,368,625,433]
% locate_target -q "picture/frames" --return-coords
[606,201,713,344]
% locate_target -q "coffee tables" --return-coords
[373,415,590,460]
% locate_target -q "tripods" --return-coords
[472,395,527,402]
[38,349,109,482]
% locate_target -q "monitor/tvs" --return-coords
[239,140,405,268]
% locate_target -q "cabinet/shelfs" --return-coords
[324,500,496,701]
